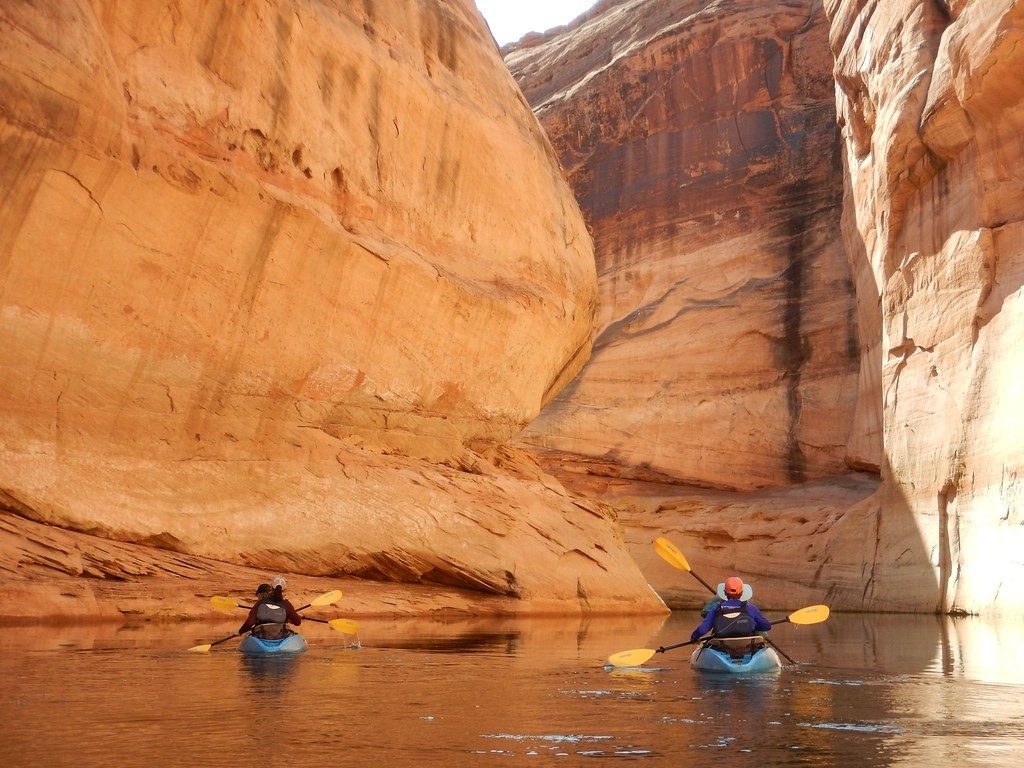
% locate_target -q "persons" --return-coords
[690,577,771,645]
[233,577,301,637]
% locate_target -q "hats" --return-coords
[256,584,271,595]
[724,577,744,595]
[718,583,753,601]
[272,577,287,591]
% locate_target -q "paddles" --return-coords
[188,589,343,652]
[653,536,798,666]
[608,605,830,668]
[209,596,361,635]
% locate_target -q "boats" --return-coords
[689,641,781,673]
[237,632,307,653]
